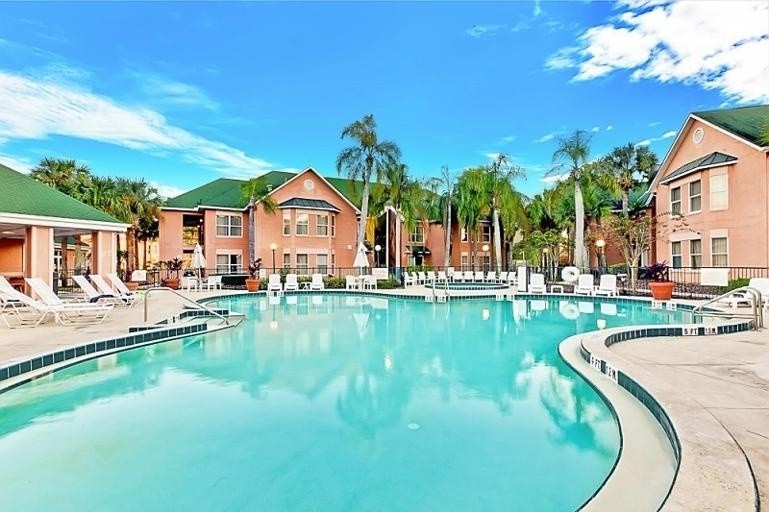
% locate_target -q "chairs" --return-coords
[528,273,547,295]
[573,274,620,297]
[267,273,324,292]
[1,272,145,329]
[345,275,377,291]
[187,275,223,293]
[719,278,769,313]
[402,270,516,289]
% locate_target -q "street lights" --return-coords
[482,245,490,276]
[542,247,548,283]
[595,240,606,284]
[270,243,278,273]
[375,245,382,268]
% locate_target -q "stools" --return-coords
[551,285,564,294]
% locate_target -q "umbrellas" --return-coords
[353,241,370,276]
[189,242,209,290]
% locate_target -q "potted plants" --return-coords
[244,257,264,292]
[163,256,185,289]
[640,259,676,300]
